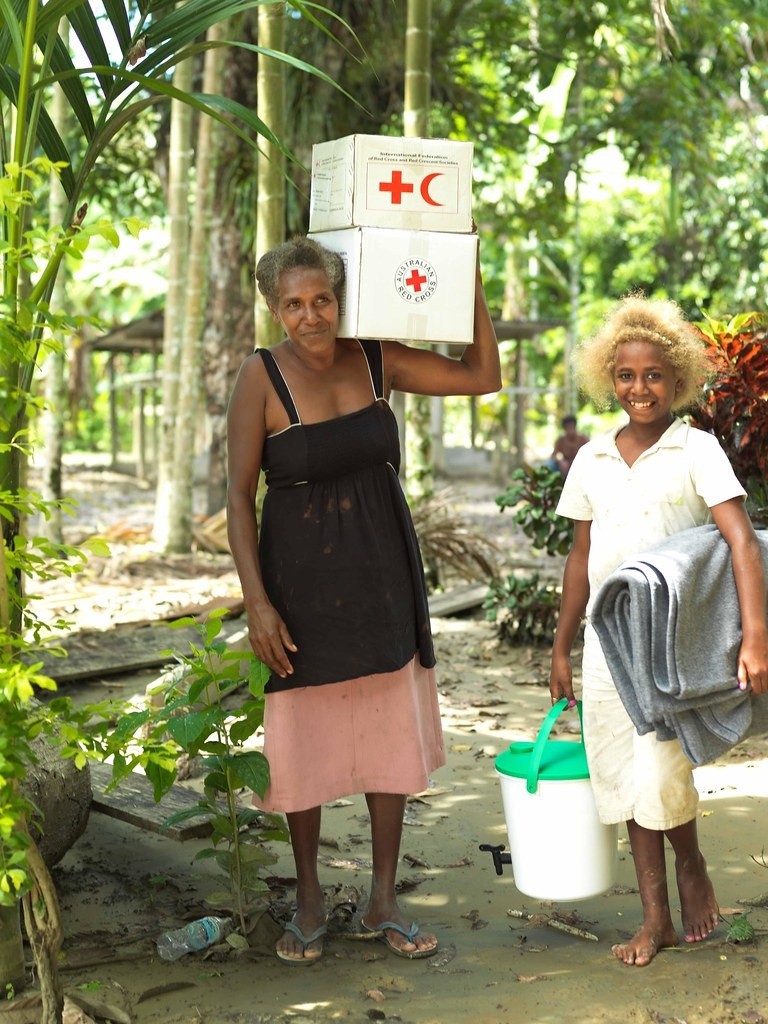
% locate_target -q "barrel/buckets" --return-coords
[494,698,618,902]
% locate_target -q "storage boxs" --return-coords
[306,227,480,345]
[310,134,474,234]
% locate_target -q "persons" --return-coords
[549,296,768,965]
[227,226,501,964]
[551,414,591,478]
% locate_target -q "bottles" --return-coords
[156,916,232,961]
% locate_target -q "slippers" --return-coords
[359,918,437,959]
[275,912,329,967]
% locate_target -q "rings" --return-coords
[551,696,560,700]
